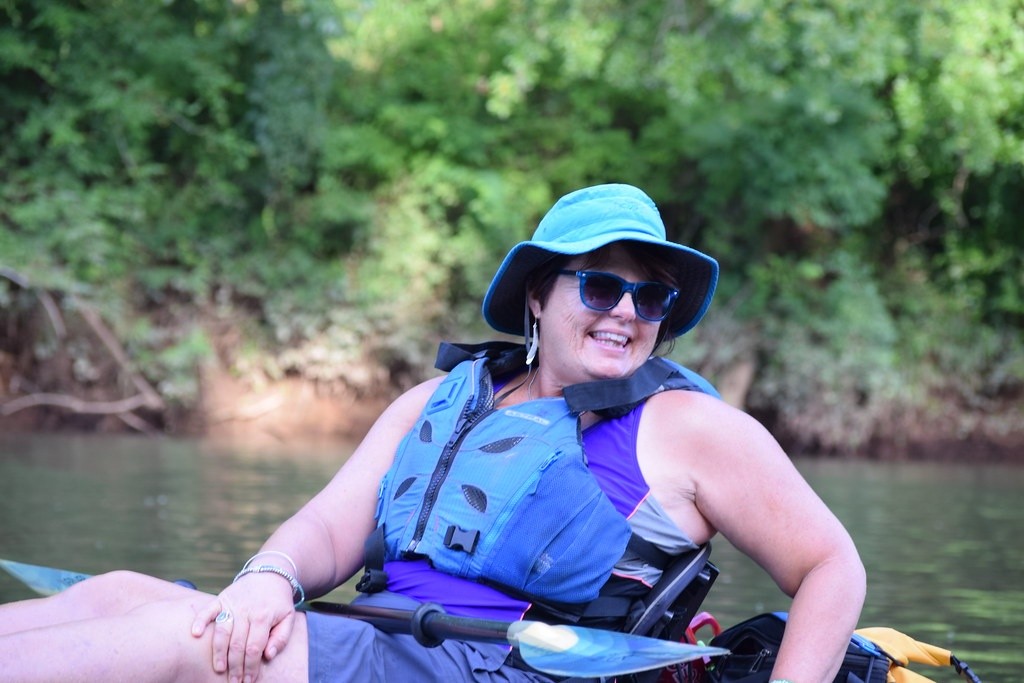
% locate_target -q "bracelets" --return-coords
[243,550,297,579]
[232,565,305,608]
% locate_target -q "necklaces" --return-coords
[529,367,538,400]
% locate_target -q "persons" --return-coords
[0,183,867,683]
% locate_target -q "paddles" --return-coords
[0,556,732,682]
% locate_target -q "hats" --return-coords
[482,184,719,341]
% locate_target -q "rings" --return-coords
[215,611,229,622]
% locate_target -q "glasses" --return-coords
[558,269,679,322]
